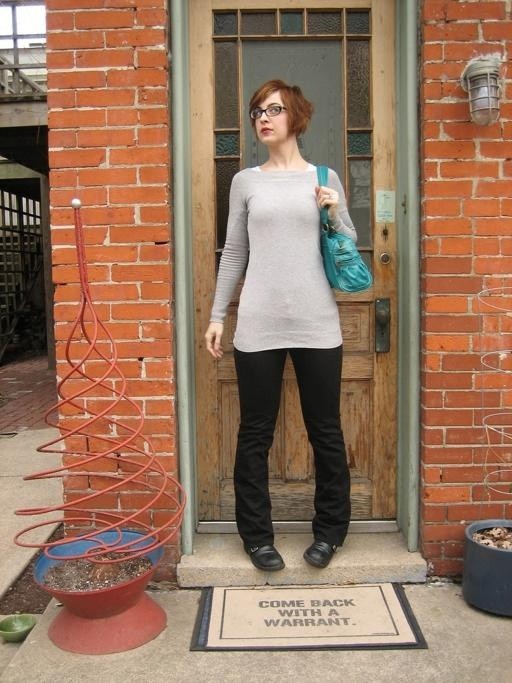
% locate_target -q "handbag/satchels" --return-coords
[322,227,371,292]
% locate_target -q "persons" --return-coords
[204,81,360,572]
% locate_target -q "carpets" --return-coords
[187,577,432,654]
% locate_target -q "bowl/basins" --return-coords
[0,614,36,645]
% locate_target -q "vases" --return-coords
[26,528,175,659]
[460,516,511,622]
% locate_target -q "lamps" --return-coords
[460,56,506,129]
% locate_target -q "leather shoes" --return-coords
[246,543,285,570]
[304,537,337,567]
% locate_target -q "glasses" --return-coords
[250,106,286,119]
